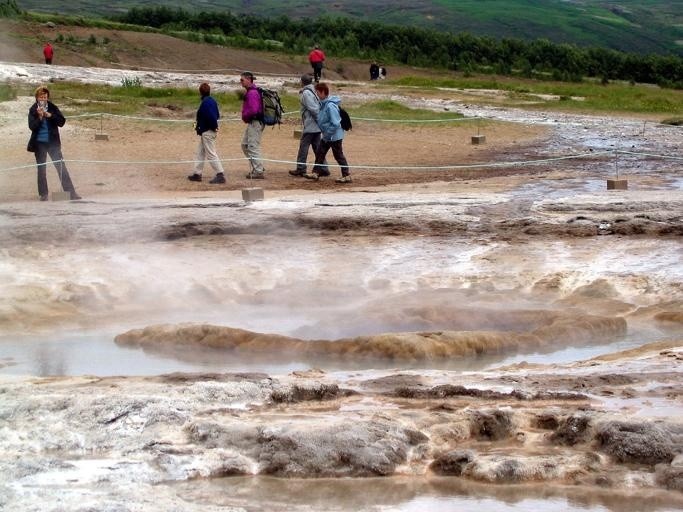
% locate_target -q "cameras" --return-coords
[38,99,48,111]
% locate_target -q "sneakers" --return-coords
[40,194,48,201]
[303,173,319,179]
[188,174,202,181]
[70,192,81,200]
[289,169,306,175]
[210,176,225,183]
[335,175,351,183]
[246,171,265,179]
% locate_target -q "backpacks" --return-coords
[338,105,352,131]
[257,87,285,125]
[381,68,386,75]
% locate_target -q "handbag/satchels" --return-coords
[321,60,326,68]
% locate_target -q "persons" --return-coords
[240,71,266,179]
[43,42,54,64]
[26,86,82,201]
[288,73,331,178]
[303,82,351,184]
[376,64,386,80]
[186,83,226,185]
[309,44,325,83]
[369,60,379,80]
